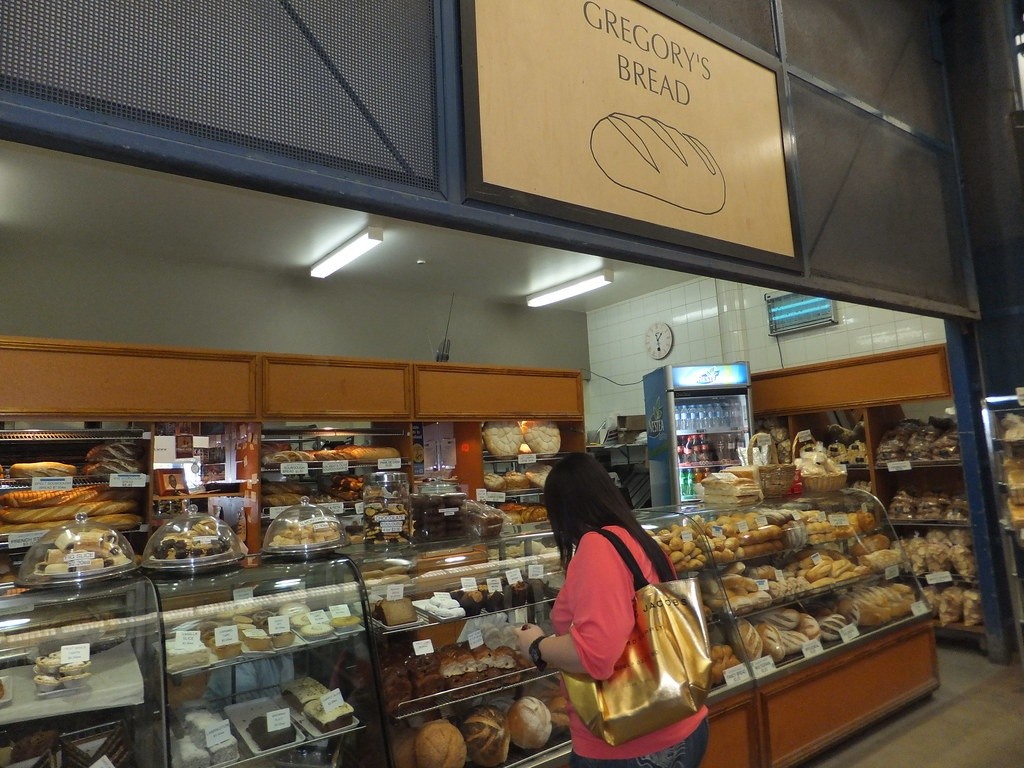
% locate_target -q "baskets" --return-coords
[791,432,848,493]
[747,433,796,499]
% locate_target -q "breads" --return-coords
[0,420,1023,768]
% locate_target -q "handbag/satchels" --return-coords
[559,527,713,747]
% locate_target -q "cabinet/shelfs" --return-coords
[0,337,1024,768]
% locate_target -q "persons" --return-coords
[167,475,184,489]
[201,580,294,706]
[513,451,710,768]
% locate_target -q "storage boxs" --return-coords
[617,414,646,444]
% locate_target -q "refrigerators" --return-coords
[642,362,755,507]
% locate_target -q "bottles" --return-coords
[677,433,744,463]
[364,472,410,552]
[681,466,726,496]
[675,403,739,429]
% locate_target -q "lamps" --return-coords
[310,225,384,278]
[526,268,614,307]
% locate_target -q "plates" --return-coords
[0,619,30,631]
[37,686,81,698]
[0,676,13,703]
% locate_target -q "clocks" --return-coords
[644,322,674,360]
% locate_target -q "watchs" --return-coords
[528,636,547,672]
